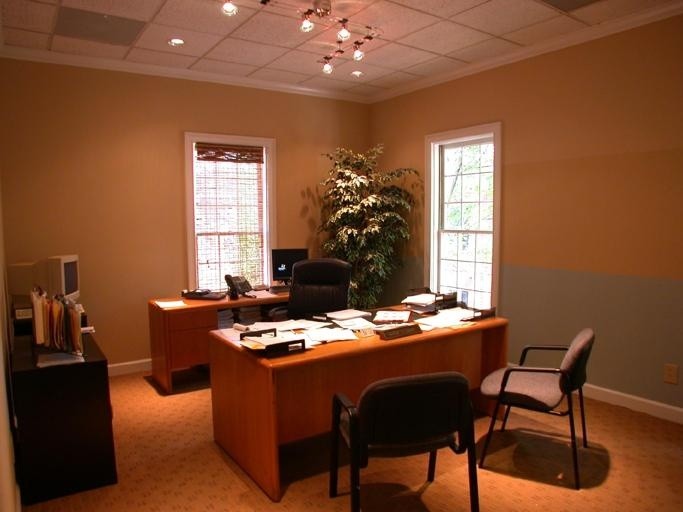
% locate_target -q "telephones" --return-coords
[225,275,252,294]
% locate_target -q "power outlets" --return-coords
[664,365,679,385]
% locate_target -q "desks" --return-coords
[145,284,289,392]
[212,297,513,503]
[5,329,120,507]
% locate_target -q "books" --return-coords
[215,290,497,355]
[152,300,187,309]
[27,285,86,368]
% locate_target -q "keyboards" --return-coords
[269,286,290,294]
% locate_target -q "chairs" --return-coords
[326,370,481,511]
[289,260,351,320]
[477,327,595,491]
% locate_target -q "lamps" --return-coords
[222,1,379,80]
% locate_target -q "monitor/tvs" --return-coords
[272,248,309,284]
[7,253,80,305]
